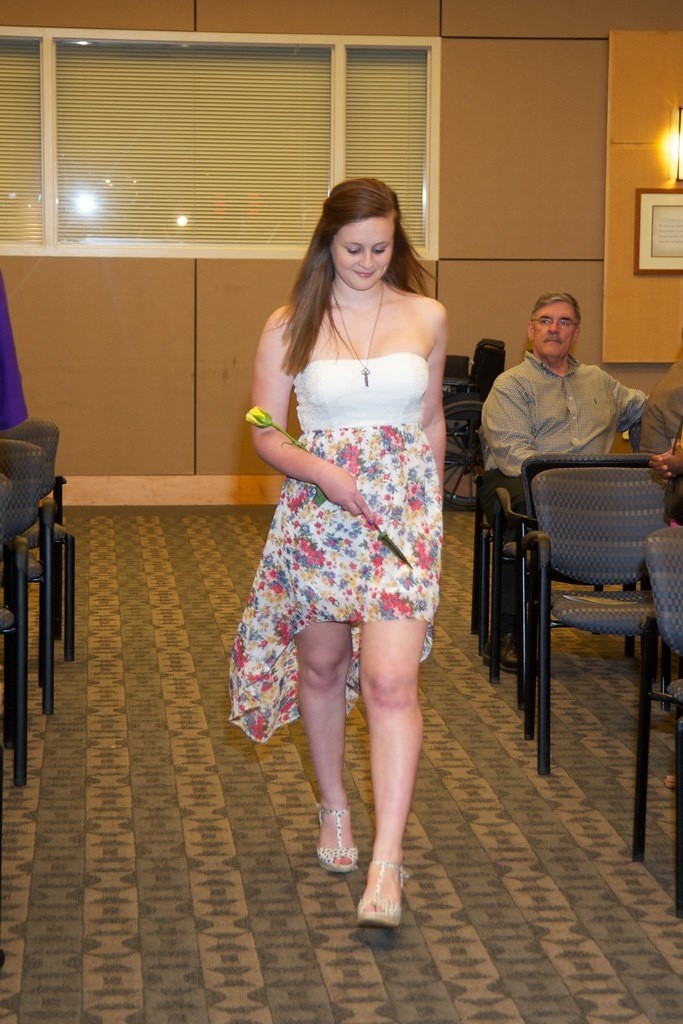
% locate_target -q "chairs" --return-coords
[0,415,77,782]
[470,427,683,915]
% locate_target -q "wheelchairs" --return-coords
[439,338,511,510]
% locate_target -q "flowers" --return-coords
[247,405,417,569]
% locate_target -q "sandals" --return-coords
[356,860,409,929]
[314,802,358,872]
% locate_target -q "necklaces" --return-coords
[331,279,383,387]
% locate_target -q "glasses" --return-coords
[531,317,577,331]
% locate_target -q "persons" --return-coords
[480,293,649,673]
[250,178,448,926]
[639,326,683,789]
